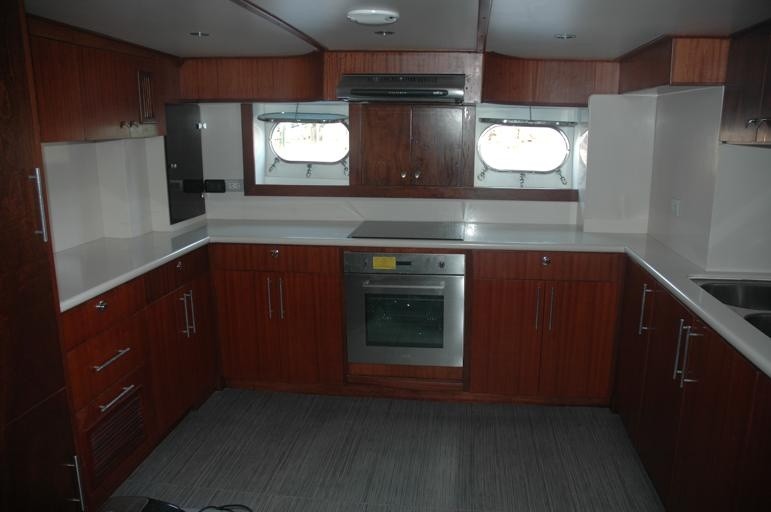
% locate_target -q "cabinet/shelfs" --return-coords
[143,242,224,437]
[0,0,85,512]
[209,243,343,395]
[616,257,656,452]
[719,23,770,148]
[469,249,626,406]
[61,272,164,512]
[30,34,167,142]
[636,285,770,511]
[349,102,475,186]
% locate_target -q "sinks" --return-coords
[744,312,771,339]
[701,281,771,311]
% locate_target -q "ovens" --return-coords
[342,254,467,369]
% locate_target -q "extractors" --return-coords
[335,72,470,108]
